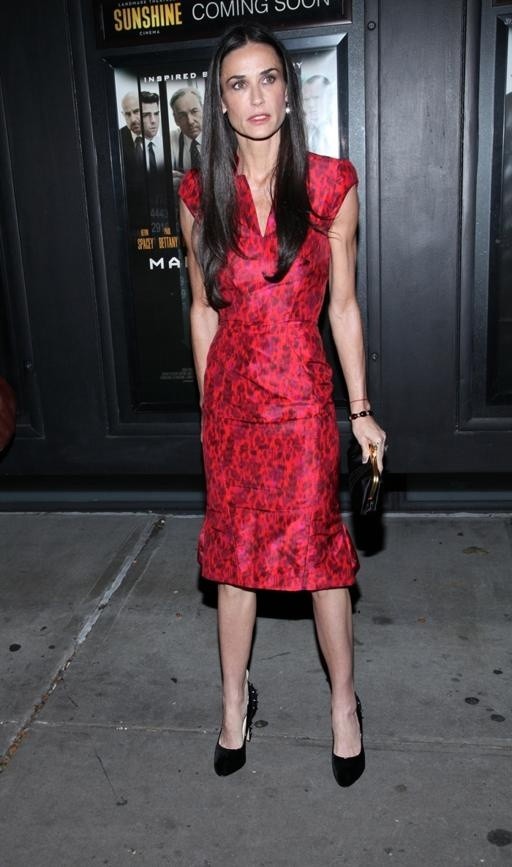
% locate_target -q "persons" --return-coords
[300,76,340,160]
[178,22,386,787]
[169,89,206,173]
[140,90,166,173]
[119,91,146,171]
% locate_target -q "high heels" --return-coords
[213,680,259,777]
[329,691,365,789]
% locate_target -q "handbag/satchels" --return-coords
[346,432,387,559]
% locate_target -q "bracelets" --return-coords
[348,398,369,404]
[348,410,376,420]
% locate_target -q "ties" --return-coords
[134,136,144,174]
[189,138,202,173]
[147,142,157,175]
